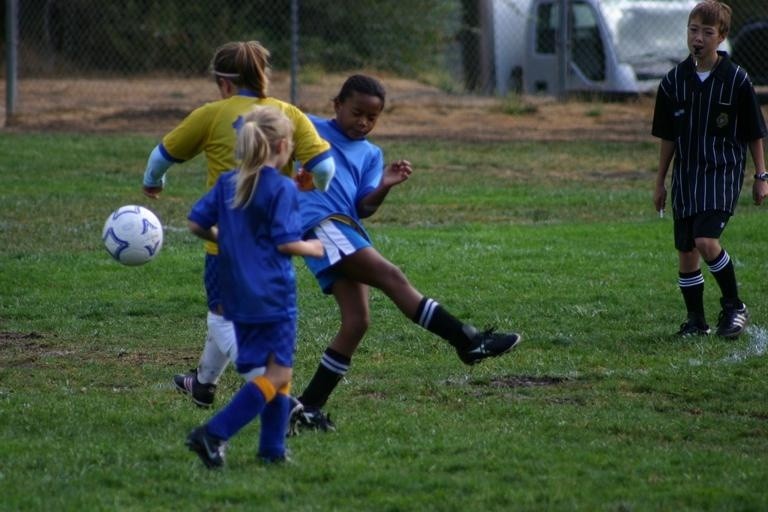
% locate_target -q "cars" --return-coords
[729,21,768,84]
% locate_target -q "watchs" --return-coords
[753,172,768,181]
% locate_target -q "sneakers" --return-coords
[184,424,229,472]
[455,323,522,367]
[667,320,711,341]
[286,394,337,437]
[172,367,217,406]
[714,301,750,339]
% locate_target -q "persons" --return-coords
[142,41,337,437]
[286,74,521,435]
[649,1,768,339]
[183,107,324,473]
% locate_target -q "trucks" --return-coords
[454,0,733,100]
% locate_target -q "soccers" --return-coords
[102,204,162,266]
